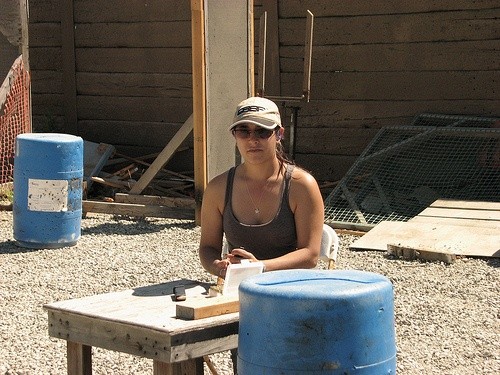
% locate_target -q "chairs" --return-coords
[221,224,338,270]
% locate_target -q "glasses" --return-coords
[233,127,278,139]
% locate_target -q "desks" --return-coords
[46,279,240,375]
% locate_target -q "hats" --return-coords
[228,97,282,130]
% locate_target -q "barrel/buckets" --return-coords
[237,268,396,375]
[13,133,83,248]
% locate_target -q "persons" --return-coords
[199,98,325,375]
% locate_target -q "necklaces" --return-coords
[244,167,276,214]
